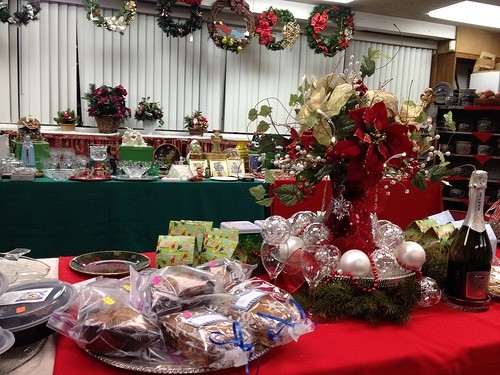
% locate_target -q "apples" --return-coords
[478,90,500,98]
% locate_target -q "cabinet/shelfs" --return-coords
[431,102,500,209]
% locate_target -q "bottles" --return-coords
[444,170,494,301]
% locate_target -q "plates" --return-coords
[153,143,181,172]
[69,250,151,276]
[433,81,454,105]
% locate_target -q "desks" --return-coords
[0,167,438,258]
[1,238,500,375]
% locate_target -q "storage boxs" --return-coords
[153,221,248,268]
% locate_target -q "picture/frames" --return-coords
[189,159,245,177]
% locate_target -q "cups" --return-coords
[478,145,489,155]
[455,140,471,155]
[48,144,107,171]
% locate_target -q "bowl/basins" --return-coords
[121,160,152,177]
[43,168,74,182]
[0,255,78,354]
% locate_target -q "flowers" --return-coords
[135,96,164,125]
[184,112,208,132]
[83,84,132,119]
[53,109,80,124]
[246,51,464,229]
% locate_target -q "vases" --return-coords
[143,119,156,135]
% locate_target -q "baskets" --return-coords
[94,115,121,133]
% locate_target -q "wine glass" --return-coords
[260,240,289,287]
[301,244,330,320]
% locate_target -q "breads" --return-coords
[83,273,291,371]
